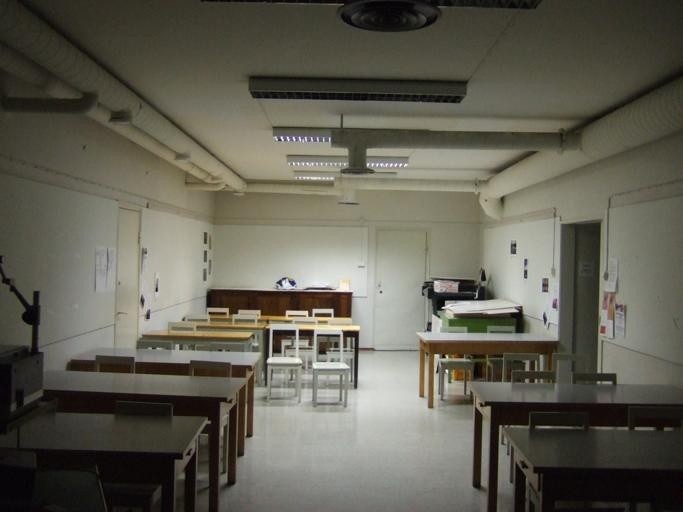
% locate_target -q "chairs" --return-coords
[95,308,350,512]
[437,326,683,512]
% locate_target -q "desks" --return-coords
[0,411,209,512]
[416,332,559,408]
[69,348,262,457]
[264,324,360,389]
[41,368,247,511]
